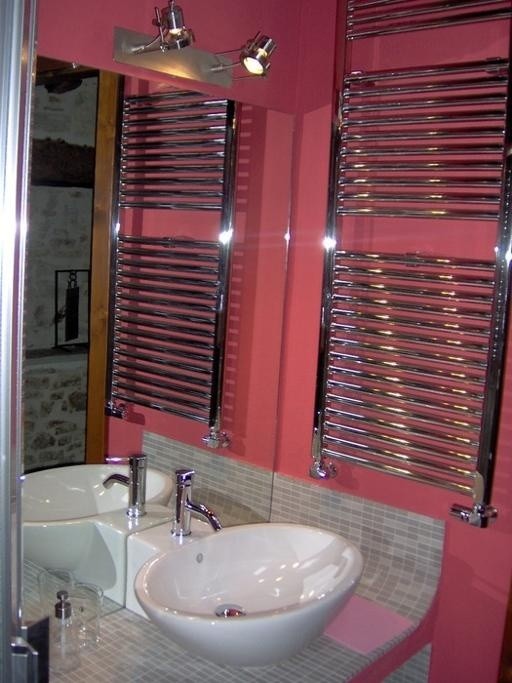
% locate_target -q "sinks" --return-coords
[125,522,365,667]
[20,464,174,610]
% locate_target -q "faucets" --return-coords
[103,452,148,518]
[175,467,224,535]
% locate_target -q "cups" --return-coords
[71,583,105,653]
[37,568,73,617]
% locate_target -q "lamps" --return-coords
[113,0,277,89]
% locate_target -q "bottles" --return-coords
[50,591,77,674]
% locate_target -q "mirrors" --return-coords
[22,56,295,634]
